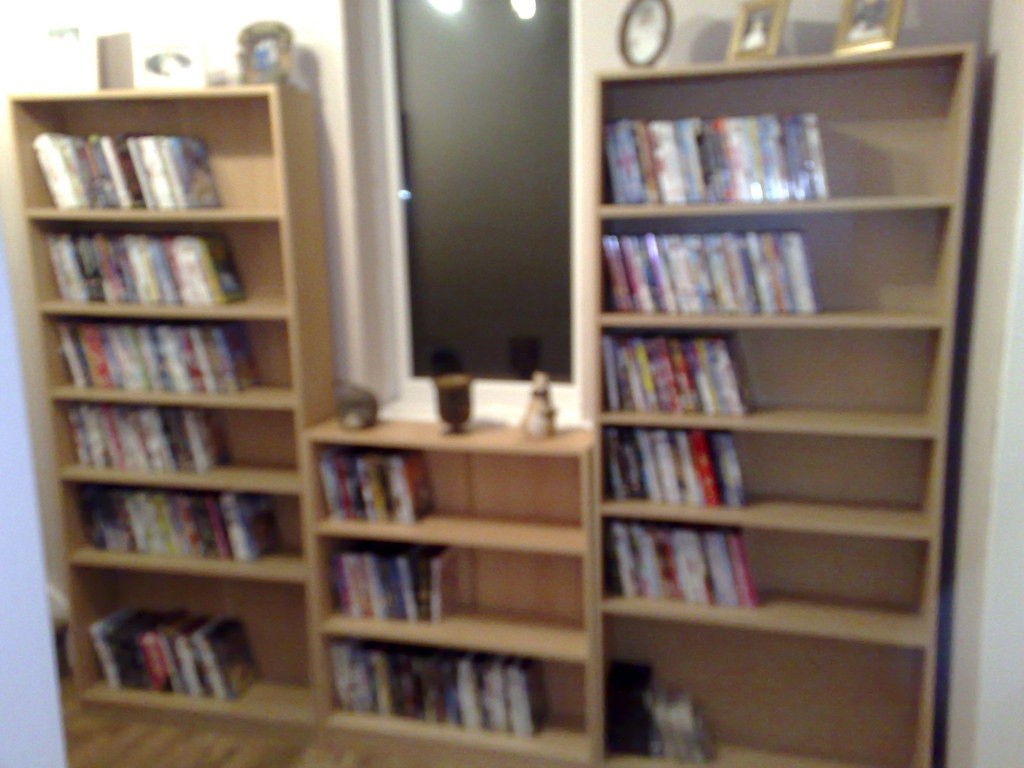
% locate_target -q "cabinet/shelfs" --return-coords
[599,42,995,768]
[2,80,331,733]
[304,415,597,768]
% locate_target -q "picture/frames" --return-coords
[617,1,904,67]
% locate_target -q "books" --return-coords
[32,133,280,702]
[648,690,717,764]
[316,447,552,740]
[602,112,831,609]
